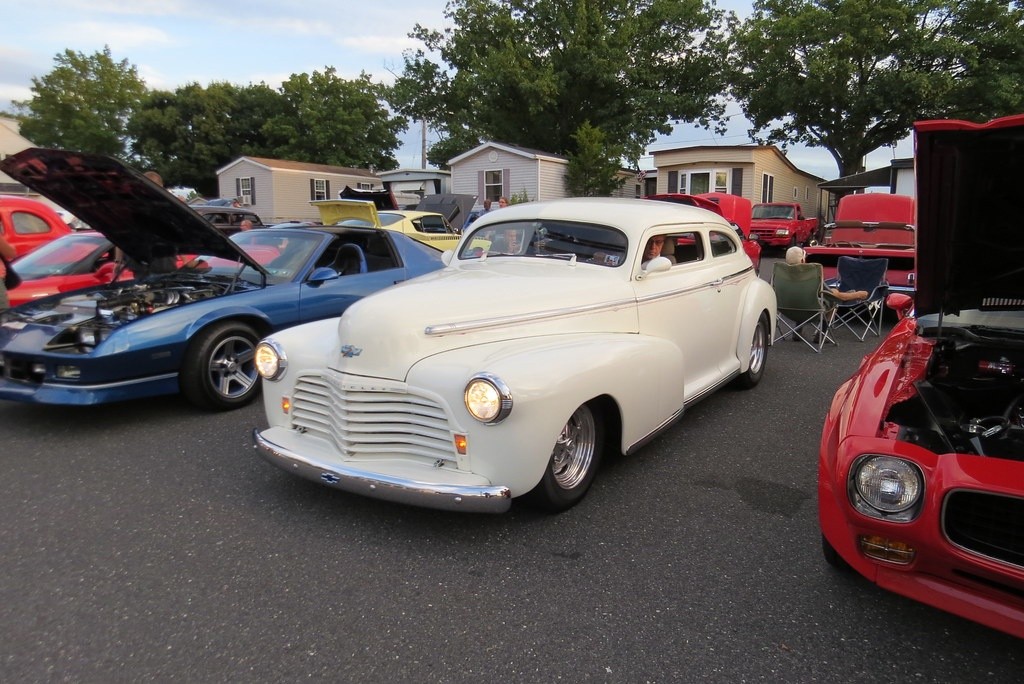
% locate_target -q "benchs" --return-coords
[674,242,730,265]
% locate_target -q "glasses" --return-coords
[648,239,665,245]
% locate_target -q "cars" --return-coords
[814,112,1024,643]
[188,197,323,238]
[4,226,281,311]
[800,191,915,291]
[0,193,93,277]
[643,192,763,277]
[309,199,492,254]
[250,195,777,515]
[0,146,483,415]
[339,184,493,233]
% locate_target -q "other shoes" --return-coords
[811,332,831,343]
[793,329,808,340]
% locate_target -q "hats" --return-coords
[785,246,806,264]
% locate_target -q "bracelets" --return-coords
[114,259,122,264]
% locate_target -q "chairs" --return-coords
[821,257,888,342]
[359,235,401,274]
[767,262,840,353]
[658,239,677,265]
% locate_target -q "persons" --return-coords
[593,233,665,269]
[479,200,495,216]
[499,197,508,207]
[490,230,516,253]
[111,171,176,279]
[785,246,868,343]
[0,236,16,310]
[240,220,253,232]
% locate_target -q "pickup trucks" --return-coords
[749,201,819,252]
